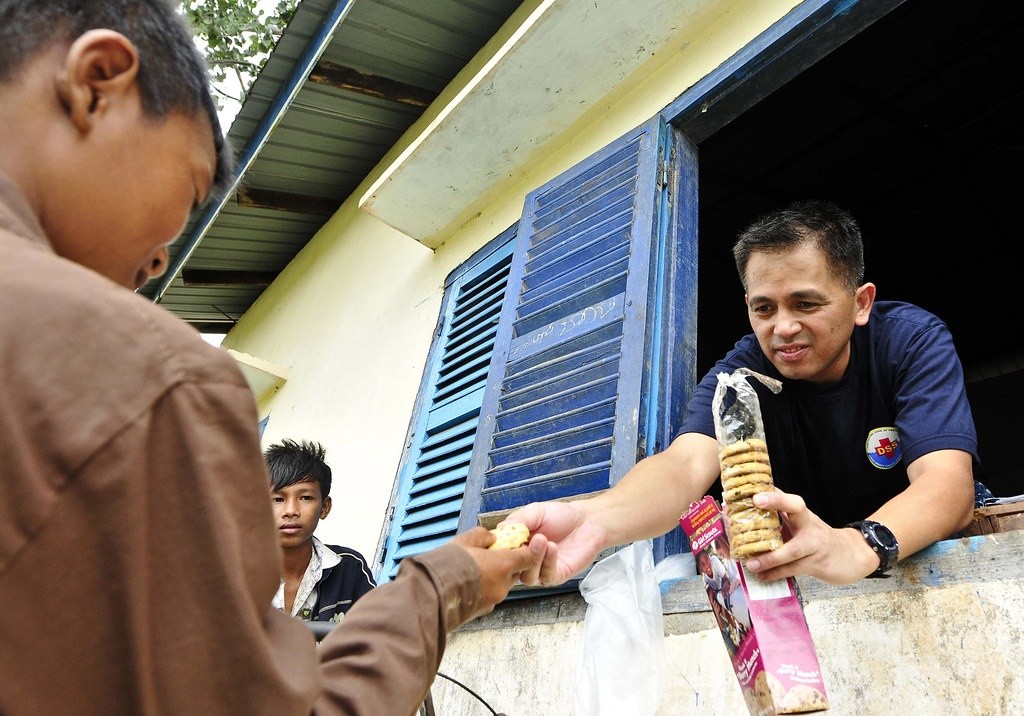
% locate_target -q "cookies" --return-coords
[743,670,829,716]
[719,439,784,557]
[485,523,530,550]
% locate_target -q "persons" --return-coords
[495,202,976,592]
[0,0,533,716]
[264,440,378,643]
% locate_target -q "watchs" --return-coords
[846,519,900,580]
